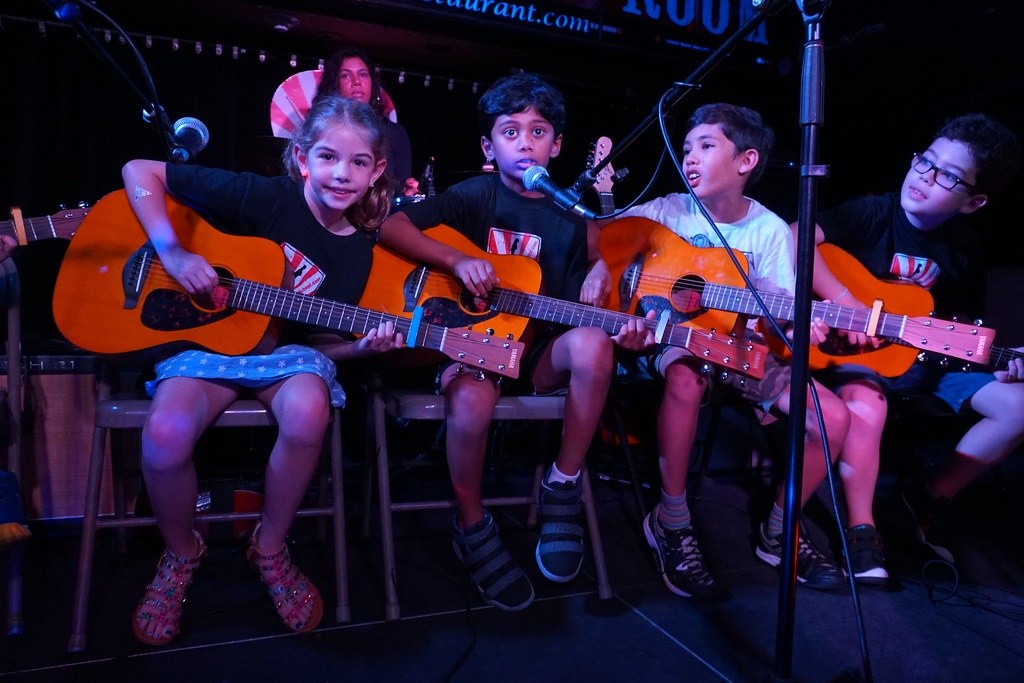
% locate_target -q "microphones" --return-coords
[523,165,597,222]
[171,117,210,162]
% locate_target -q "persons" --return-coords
[789,113,1024,584]
[311,49,425,208]
[378,76,654,611]
[579,103,851,598]
[121,96,403,644]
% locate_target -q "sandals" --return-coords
[134,530,208,644]
[247,521,325,632]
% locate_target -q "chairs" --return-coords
[65,348,351,651]
[364,378,613,621]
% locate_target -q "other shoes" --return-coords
[0,470,31,544]
[901,480,960,563]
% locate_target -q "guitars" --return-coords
[352,224,771,389]
[760,242,1024,379]
[51,187,527,390]
[599,216,996,375]
[583,134,620,218]
[1,199,100,246]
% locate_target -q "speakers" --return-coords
[0,353,123,527]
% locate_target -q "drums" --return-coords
[387,193,428,213]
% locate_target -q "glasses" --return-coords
[913,151,978,194]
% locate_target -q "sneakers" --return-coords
[447,507,536,610]
[642,504,705,597]
[837,523,888,578]
[535,466,587,581]
[756,523,847,588]
